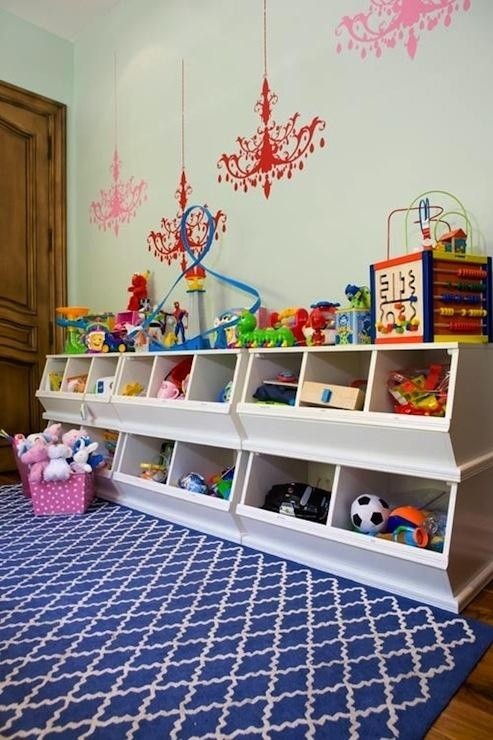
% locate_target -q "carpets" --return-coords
[0,483,491,740]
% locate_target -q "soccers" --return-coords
[351,494,391,533]
[387,506,427,534]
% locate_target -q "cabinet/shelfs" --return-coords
[33,341,493,615]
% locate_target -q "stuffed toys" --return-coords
[11,422,106,484]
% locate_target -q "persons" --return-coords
[160,301,189,345]
